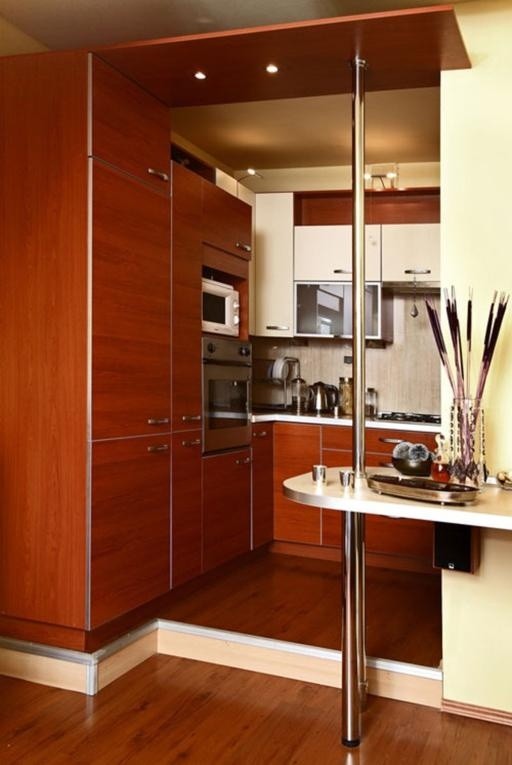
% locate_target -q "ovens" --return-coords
[201,335,253,454]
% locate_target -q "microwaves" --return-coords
[201,276,239,337]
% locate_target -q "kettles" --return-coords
[306,380,338,413]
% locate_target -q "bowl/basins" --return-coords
[392,458,434,475]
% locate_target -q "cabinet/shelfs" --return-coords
[0,50,172,652]
[192,165,255,336]
[273,423,439,575]
[251,423,274,559]
[294,282,393,343]
[294,223,440,286]
[251,191,293,338]
[173,162,251,602]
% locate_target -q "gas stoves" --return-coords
[379,411,442,426]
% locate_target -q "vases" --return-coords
[449,402,484,492]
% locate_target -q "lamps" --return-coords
[363,172,396,180]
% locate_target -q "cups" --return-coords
[312,465,327,486]
[340,470,353,489]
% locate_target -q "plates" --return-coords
[368,474,479,504]
